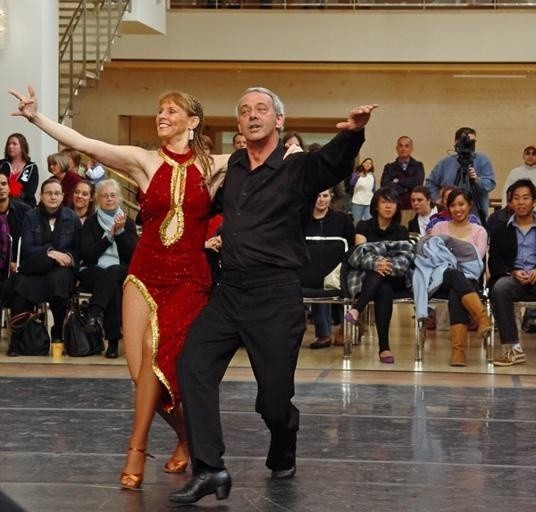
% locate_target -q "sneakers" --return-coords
[311,338,332,349]
[380,356,394,364]
[343,314,358,324]
[493,348,526,367]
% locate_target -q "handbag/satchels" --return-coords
[65,312,104,356]
[8,313,56,356]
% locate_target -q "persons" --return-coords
[167,86,378,504]
[8,85,304,489]
[286,127,536,366]
[0,134,140,360]
[202,132,247,278]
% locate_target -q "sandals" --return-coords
[164,456,188,474]
[120,447,146,489]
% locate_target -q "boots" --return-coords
[450,324,468,366]
[462,292,493,337]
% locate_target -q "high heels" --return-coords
[168,469,231,503]
[271,465,297,479]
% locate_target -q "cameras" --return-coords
[454,130,476,152]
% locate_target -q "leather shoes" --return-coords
[106,344,118,358]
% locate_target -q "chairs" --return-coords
[302,229,535,364]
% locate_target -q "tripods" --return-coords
[454,153,486,225]
